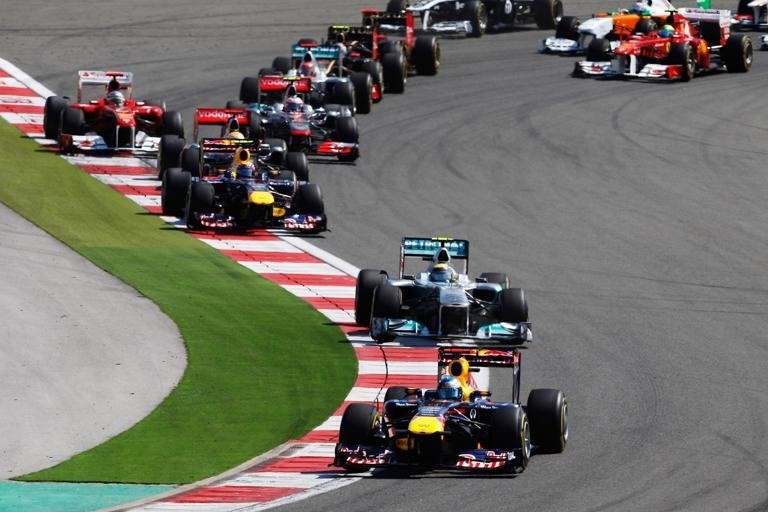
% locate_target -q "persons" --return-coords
[656,23,673,35]
[424,263,452,285]
[227,128,245,142]
[280,94,305,111]
[436,373,461,403]
[103,88,128,102]
[233,161,258,181]
[301,60,320,79]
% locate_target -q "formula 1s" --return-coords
[330,342,568,479]
[219,0,439,162]
[352,237,531,348]
[542,0,767,82]
[158,108,329,238]
[39,69,185,154]
[388,0,562,37]
